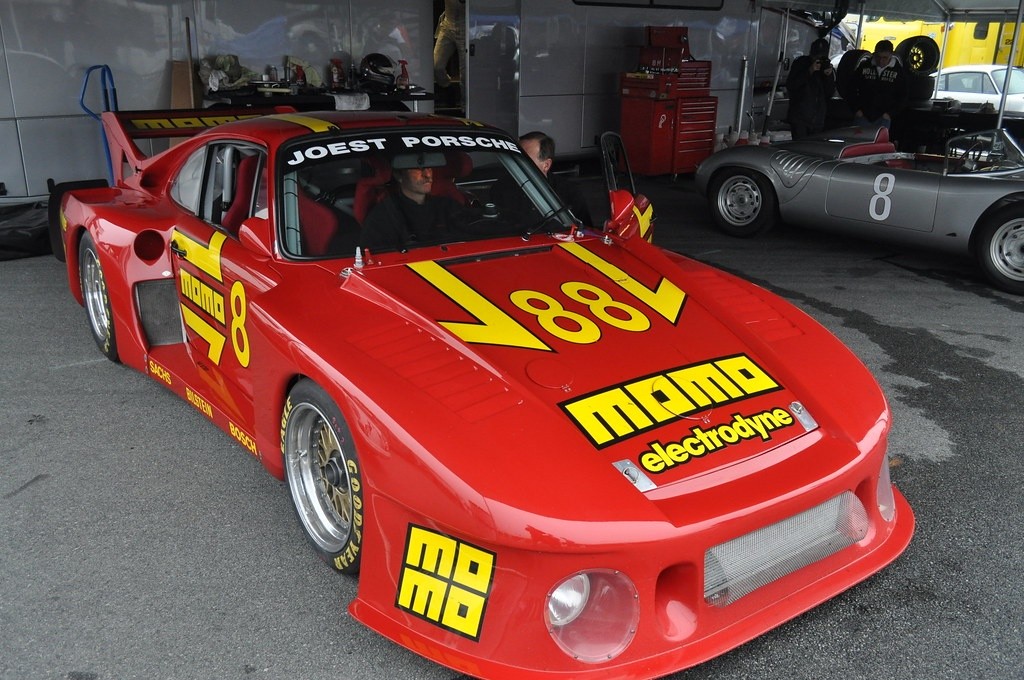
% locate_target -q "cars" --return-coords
[59,108,916,679]
[929,64,1024,112]
[694,126,1024,295]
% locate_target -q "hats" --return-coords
[811,39,829,56]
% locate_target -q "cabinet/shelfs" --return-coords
[618,97,676,184]
[671,96,718,185]
[675,61,712,99]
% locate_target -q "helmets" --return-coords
[360,53,397,84]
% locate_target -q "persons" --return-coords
[846,39,906,130]
[359,163,480,254]
[433,0,466,118]
[481,129,592,236]
[784,38,837,140]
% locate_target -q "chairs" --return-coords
[219,150,467,258]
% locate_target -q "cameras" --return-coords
[817,57,831,71]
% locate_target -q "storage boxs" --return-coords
[615,72,677,100]
